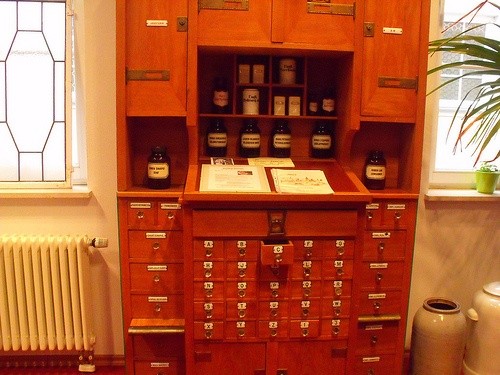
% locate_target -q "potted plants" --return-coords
[475,161,500,194]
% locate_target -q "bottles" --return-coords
[279,58,297,83]
[146,148,171,189]
[308,88,336,116]
[270,119,291,157]
[237,58,252,83]
[211,77,232,113]
[311,121,334,158]
[410,296,466,375]
[288,89,302,116]
[364,150,386,190]
[242,88,260,116]
[239,118,261,158]
[273,88,288,116]
[205,118,227,157]
[252,58,266,83]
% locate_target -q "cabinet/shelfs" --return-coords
[116,0,430,375]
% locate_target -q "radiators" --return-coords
[0,235,108,372]
